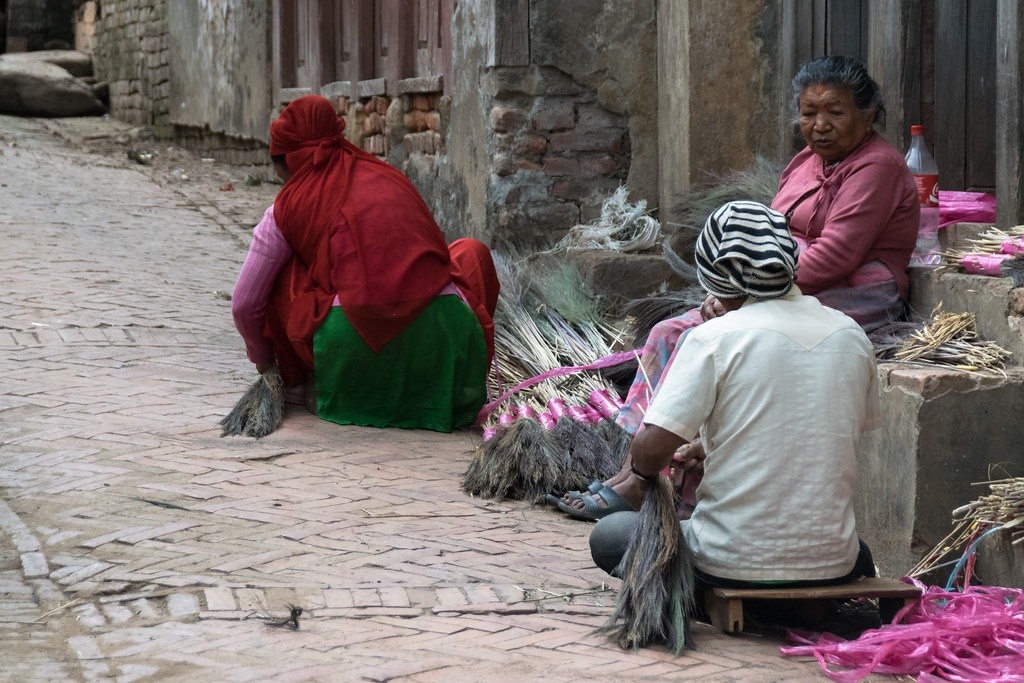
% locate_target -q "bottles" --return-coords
[902,125,942,266]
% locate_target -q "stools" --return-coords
[705,578,922,633]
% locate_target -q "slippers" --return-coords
[546,478,604,503]
[558,486,634,518]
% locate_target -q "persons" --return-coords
[586,201,878,626]
[546,54,921,522]
[231,97,500,432]
